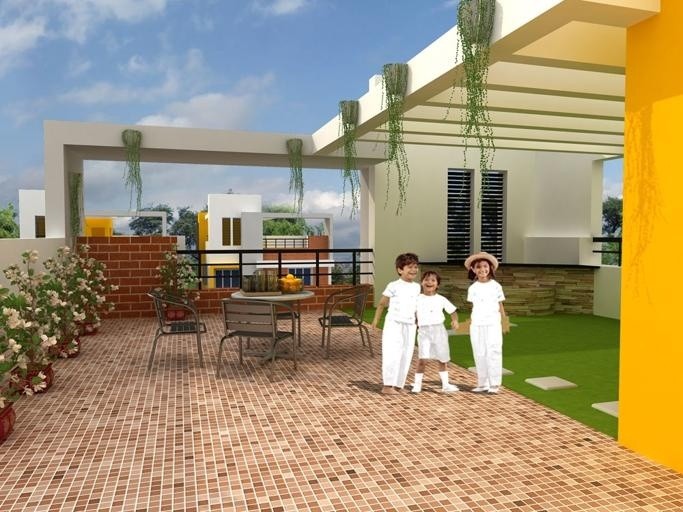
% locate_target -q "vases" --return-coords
[164,306,184,319]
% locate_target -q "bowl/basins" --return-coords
[277,278,304,293]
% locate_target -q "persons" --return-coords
[464,251,510,393]
[371,252,421,395]
[411,271,460,393]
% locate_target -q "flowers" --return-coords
[0,243,119,443]
[148,240,203,310]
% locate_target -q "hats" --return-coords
[464,252,499,270]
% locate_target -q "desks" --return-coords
[229,288,314,365]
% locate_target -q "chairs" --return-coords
[214,298,298,382]
[144,291,209,370]
[316,283,377,360]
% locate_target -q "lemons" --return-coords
[282,274,301,291]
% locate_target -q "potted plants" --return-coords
[67,169,83,239]
[119,128,145,219]
[336,97,362,222]
[441,1,495,210]
[378,61,410,218]
[285,137,304,218]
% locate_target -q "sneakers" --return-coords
[471,387,499,393]
